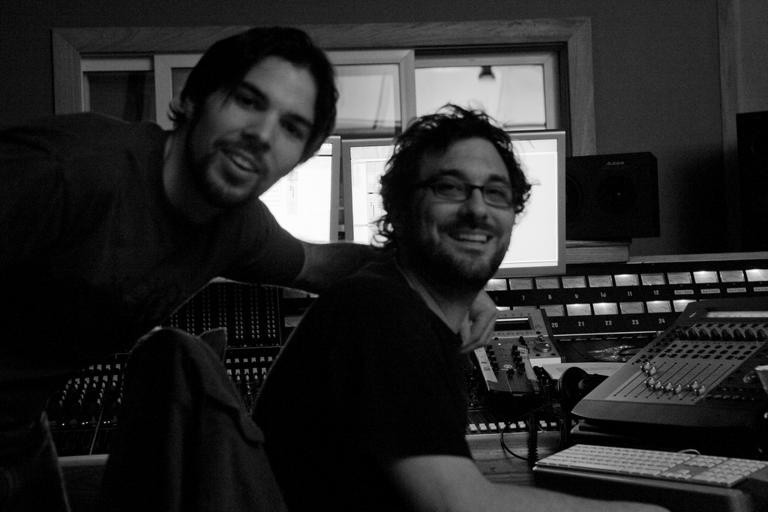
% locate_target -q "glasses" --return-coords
[415,174,524,214]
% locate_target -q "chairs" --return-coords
[107,327,288,512]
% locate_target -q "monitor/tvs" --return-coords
[340,130,566,278]
[210,135,341,283]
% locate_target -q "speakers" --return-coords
[566,150,660,240]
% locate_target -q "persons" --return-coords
[1,24,498,512]
[253,99,673,512]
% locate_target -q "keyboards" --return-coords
[534,444,768,488]
